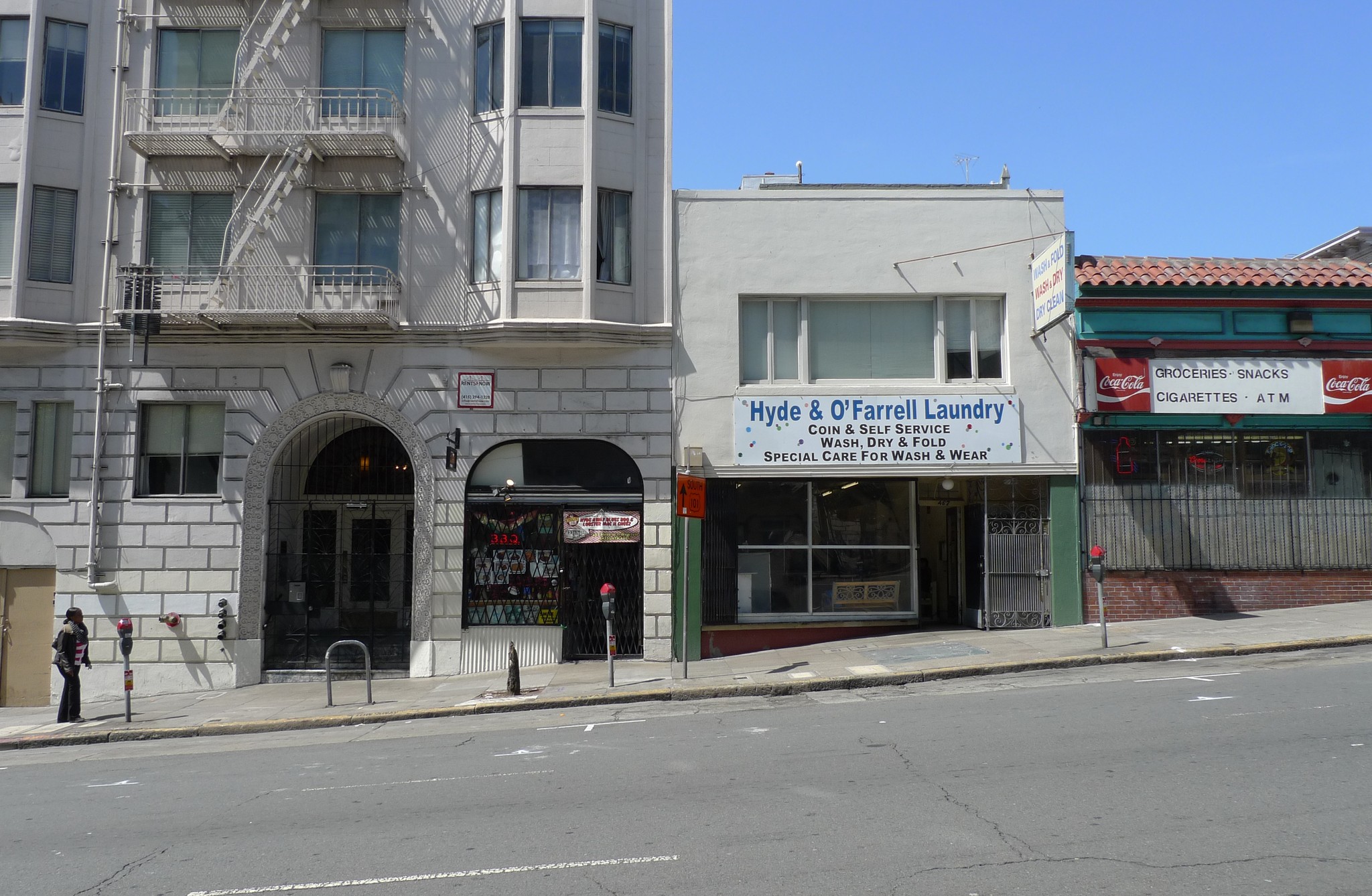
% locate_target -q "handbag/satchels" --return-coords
[51,638,58,650]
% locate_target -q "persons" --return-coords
[52,607,92,723]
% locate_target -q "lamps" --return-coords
[821,490,832,497]
[841,481,859,489]
[942,476,954,490]
[493,479,514,501]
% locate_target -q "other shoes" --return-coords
[69,716,86,723]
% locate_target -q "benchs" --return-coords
[822,580,900,612]
